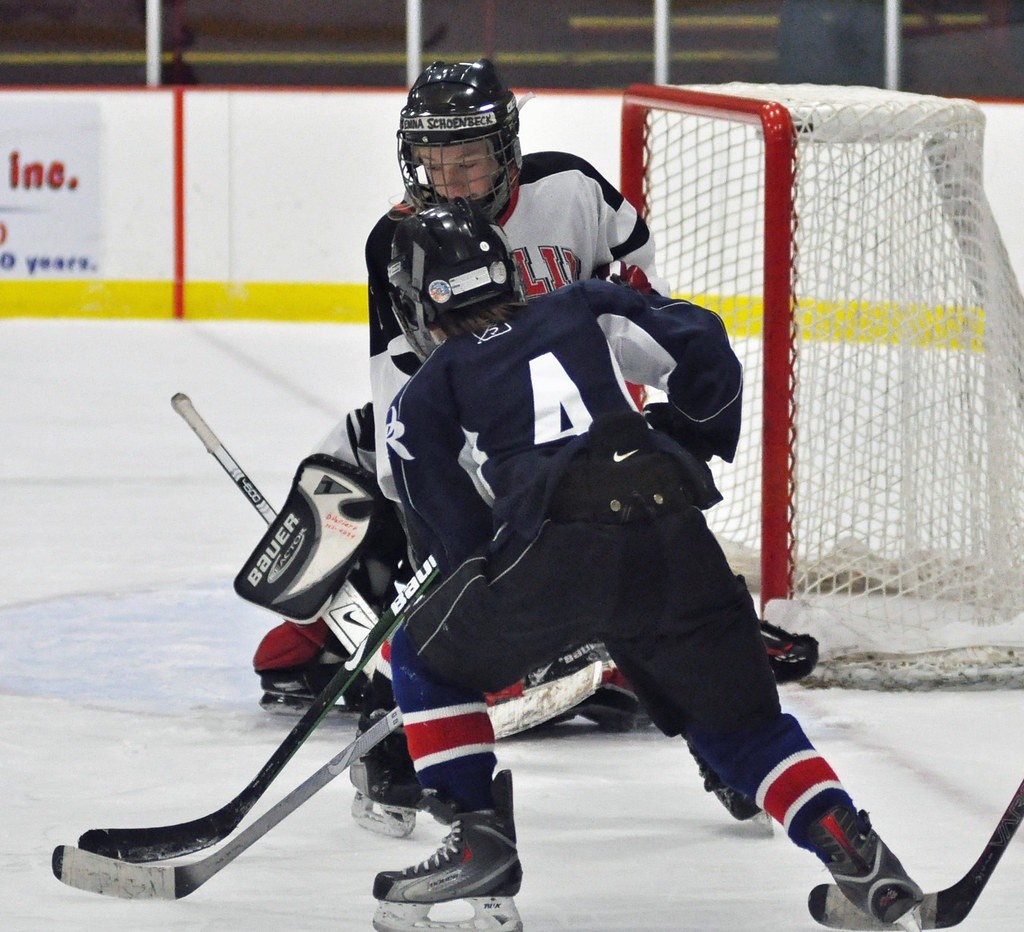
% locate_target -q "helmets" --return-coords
[385,200,515,364]
[398,57,523,222]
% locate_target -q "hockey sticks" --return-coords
[75,549,444,866]
[805,781,1024,932]
[49,702,399,904]
[162,390,612,749]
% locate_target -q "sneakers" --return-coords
[688,748,776,839]
[806,805,926,932]
[256,666,360,721]
[345,717,425,836]
[372,768,522,932]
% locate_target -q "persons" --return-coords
[253,60,821,719]
[372,204,925,932]
